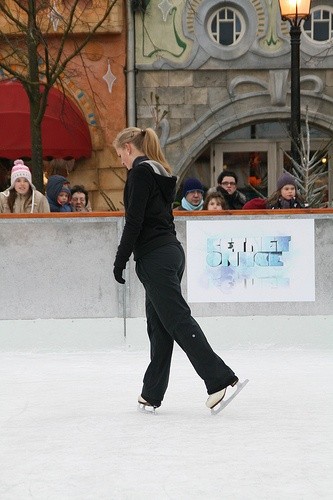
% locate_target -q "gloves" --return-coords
[113,267,125,284]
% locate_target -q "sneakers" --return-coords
[206,376,249,415]
[137,396,161,413]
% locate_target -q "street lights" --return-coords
[278,0,311,208]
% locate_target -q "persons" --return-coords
[113,127,249,416]
[0,158,303,214]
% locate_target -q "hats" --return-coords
[57,184,72,207]
[277,173,297,192]
[241,198,268,209]
[183,177,204,198]
[1,159,36,213]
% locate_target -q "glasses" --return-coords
[221,181,237,186]
